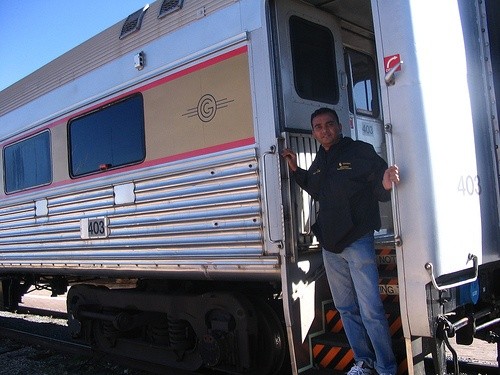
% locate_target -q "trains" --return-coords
[0,1,500,375]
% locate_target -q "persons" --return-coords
[280,107,400,375]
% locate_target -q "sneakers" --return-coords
[346,360,375,375]
